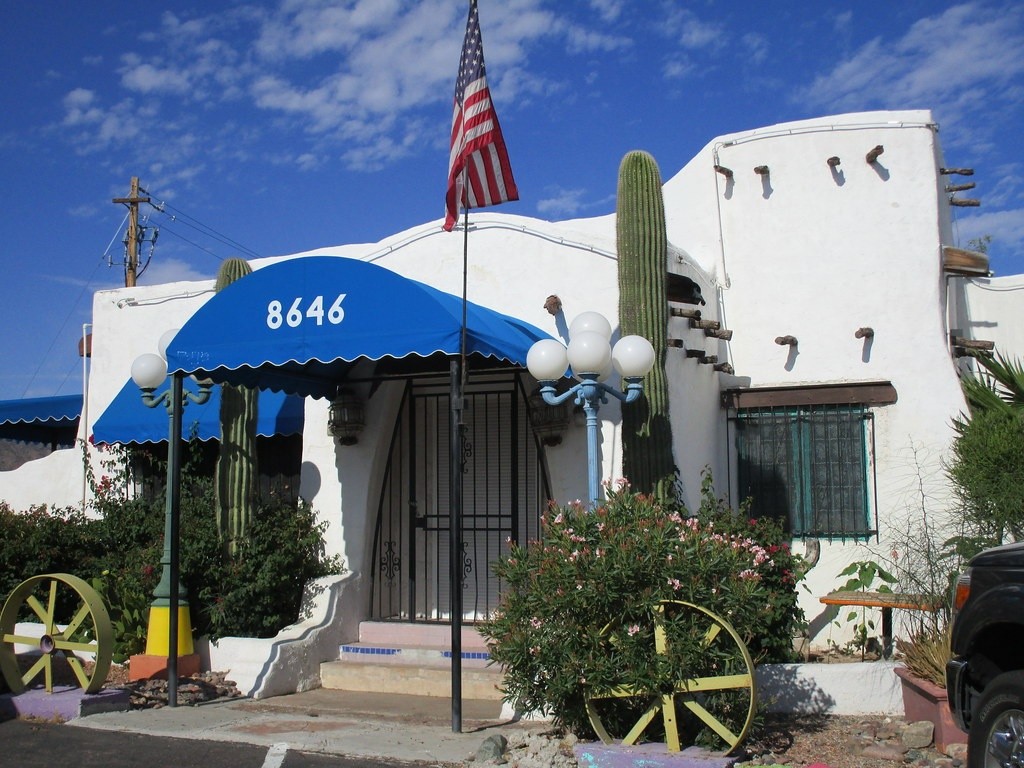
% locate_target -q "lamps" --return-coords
[329,385,365,447]
[527,388,570,447]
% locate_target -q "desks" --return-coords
[820,590,946,659]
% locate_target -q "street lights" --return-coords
[525,310,656,513]
[128,330,218,654]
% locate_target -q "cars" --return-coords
[946,540,1024,768]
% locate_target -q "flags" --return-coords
[444,0,518,232]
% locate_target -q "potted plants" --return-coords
[893,624,968,755]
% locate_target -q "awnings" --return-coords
[92,367,305,443]
[166,256,574,730]
[0,393,84,452]
